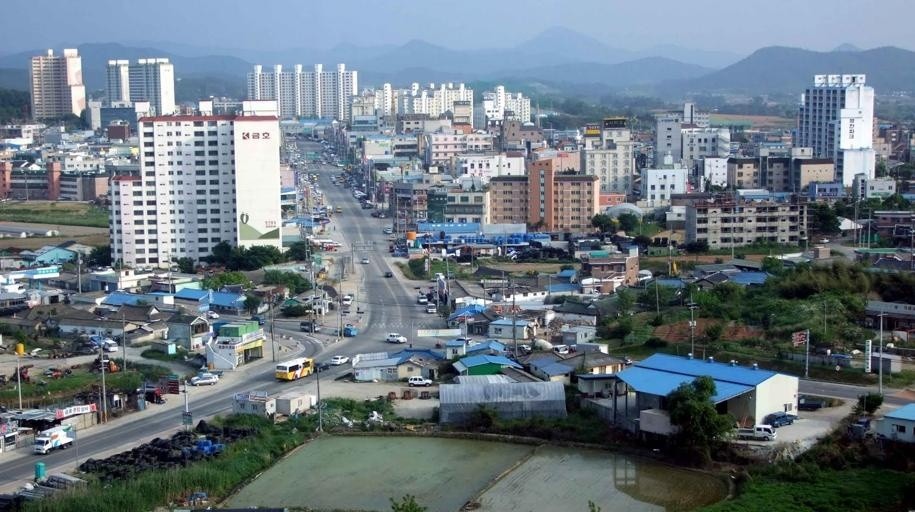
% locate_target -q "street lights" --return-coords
[15,358,23,411]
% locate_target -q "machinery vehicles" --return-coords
[532,337,569,355]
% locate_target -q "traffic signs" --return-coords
[792,330,808,347]
[158,375,179,395]
[182,412,192,425]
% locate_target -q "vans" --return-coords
[98,352,110,363]
[812,342,837,355]
[823,354,854,367]
[300,320,320,332]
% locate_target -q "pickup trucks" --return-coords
[798,395,823,409]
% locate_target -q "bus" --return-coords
[276,357,314,381]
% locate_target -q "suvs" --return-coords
[384,333,408,343]
[408,375,432,386]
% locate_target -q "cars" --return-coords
[286,139,386,219]
[520,344,531,350]
[450,336,473,346]
[135,265,153,272]
[385,271,392,277]
[363,257,369,264]
[331,355,350,366]
[617,355,633,365]
[383,226,393,234]
[171,265,178,272]
[819,238,830,244]
[314,362,330,371]
[190,372,219,386]
[208,310,220,319]
[417,295,428,303]
[207,270,215,278]
[343,295,352,306]
[426,304,436,313]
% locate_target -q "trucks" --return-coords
[245,316,263,325]
[33,425,75,456]
[90,335,119,352]
[734,425,776,441]
[768,412,797,427]
[336,323,359,337]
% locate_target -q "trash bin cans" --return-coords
[35,462,45,481]
